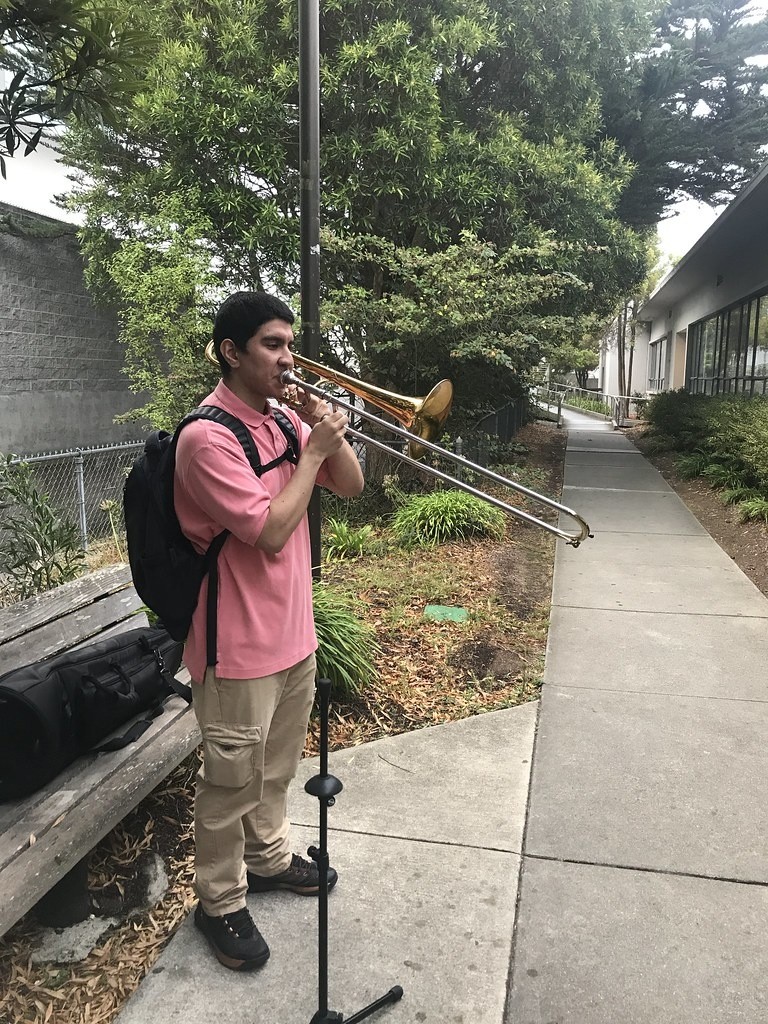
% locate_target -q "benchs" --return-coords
[0,562,205,937]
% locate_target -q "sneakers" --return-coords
[246,852,338,896]
[194,901,271,971]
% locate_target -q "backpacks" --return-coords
[123,406,300,640]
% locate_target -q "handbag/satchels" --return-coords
[0,626,192,804]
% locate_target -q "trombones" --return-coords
[204,335,591,549]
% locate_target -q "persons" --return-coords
[173,293,365,973]
